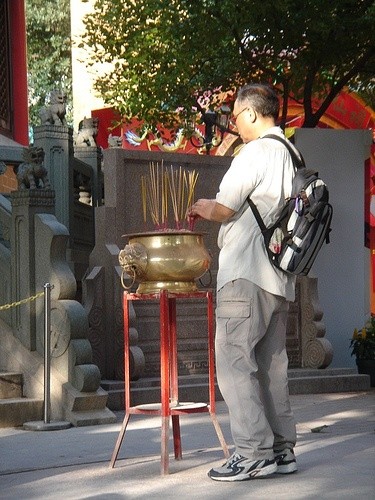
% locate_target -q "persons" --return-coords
[185,83,302,481]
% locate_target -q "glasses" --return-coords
[230,107,247,128]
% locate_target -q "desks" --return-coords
[112,290,233,482]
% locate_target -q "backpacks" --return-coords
[243,134,333,276]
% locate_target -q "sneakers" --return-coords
[208,451,278,482]
[273,448,298,473]
